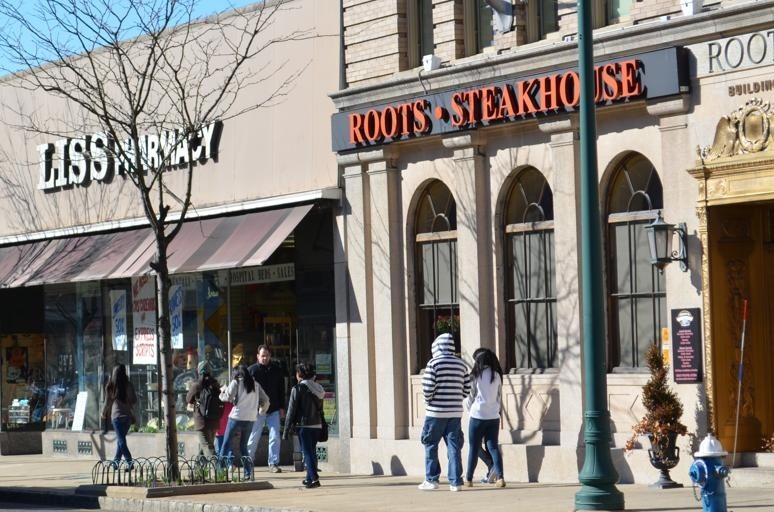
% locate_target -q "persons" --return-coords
[282,361,327,489]
[216,363,270,479]
[216,383,237,472]
[417,332,471,493]
[96,364,137,474]
[464,350,506,487]
[246,341,285,474]
[473,346,499,485]
[183,360,221,476]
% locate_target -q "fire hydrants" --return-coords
[690,433,730,511]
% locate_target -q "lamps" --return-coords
[643,208,689,275]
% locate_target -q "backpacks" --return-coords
[194,380,225,420]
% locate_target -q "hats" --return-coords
[198,360,212,375]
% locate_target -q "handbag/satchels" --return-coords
[317,415,328,442]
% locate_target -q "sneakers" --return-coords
[267,464,281,473]
[302,480,320,488]
[418,480,439,489]
[450,466,506,491]
